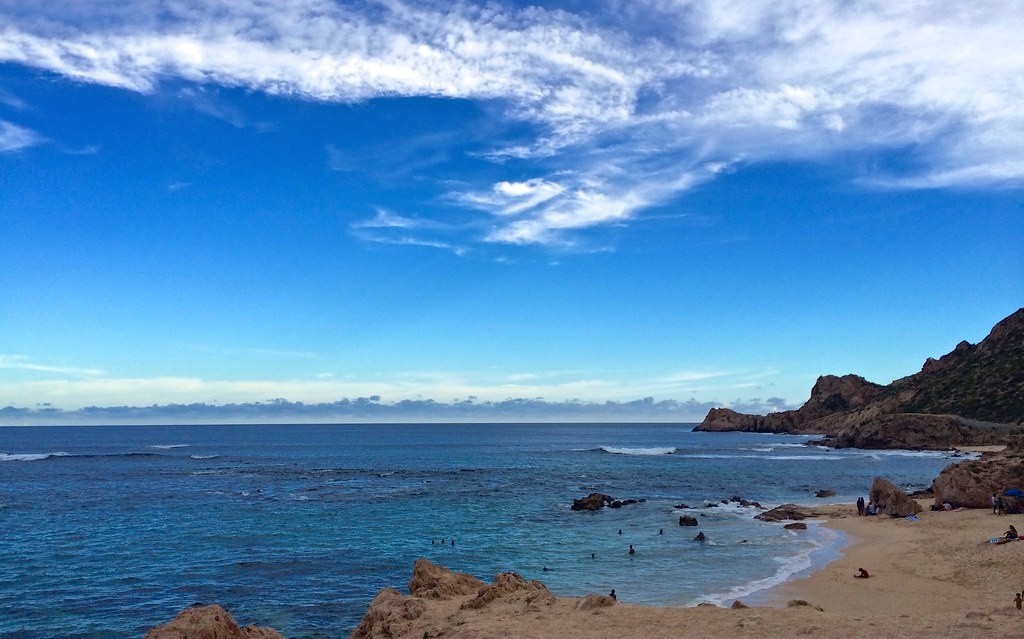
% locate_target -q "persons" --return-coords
[991,493,1020,516]
[1004,525,1018,540]
[929,501,952,511]
[628,545,635,554]
[609,589,616,601]
[857,497,875,516]
[853,568,868,578]
[1013,593,1022,610]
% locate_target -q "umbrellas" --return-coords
[1005,489,1024,498]
